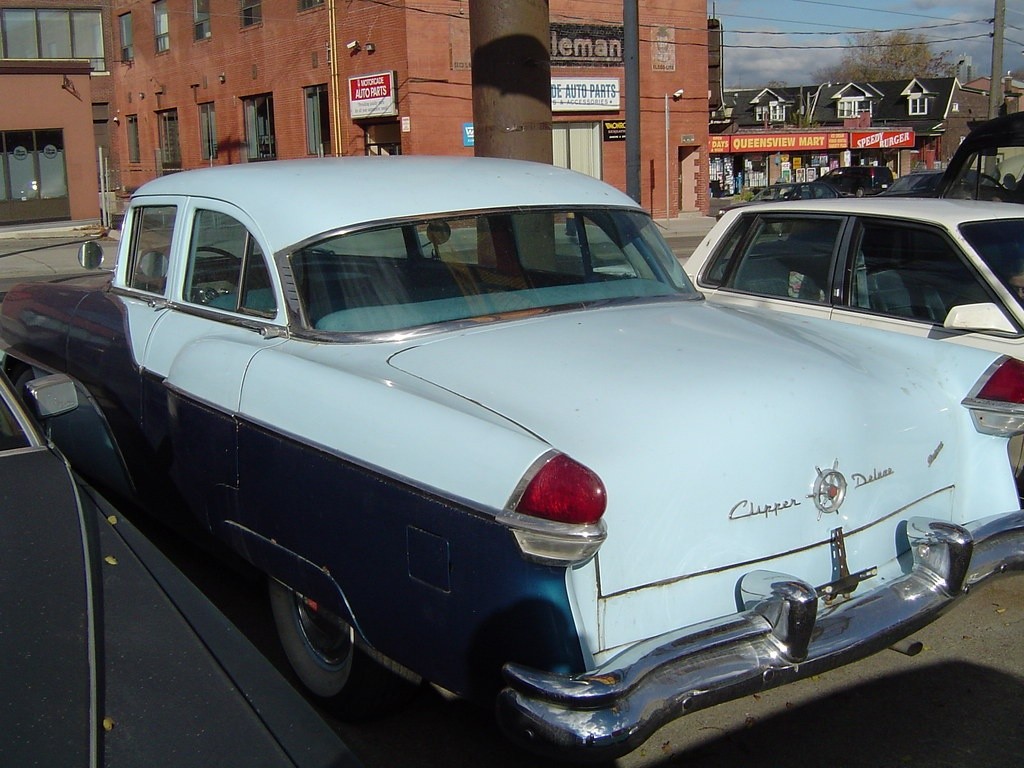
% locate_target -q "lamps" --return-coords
[366,43,374,50]
[219,73,225,83]
[139,93,144,101]
[951,102,960,115]
[61,74,67,90]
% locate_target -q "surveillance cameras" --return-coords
[346,41,358,49]
[113,117,119,122]
[673,90,683,99]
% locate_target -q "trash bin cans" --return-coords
[776,178,787,191]
[710,180,721,198]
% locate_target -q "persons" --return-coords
[1003,174,1024,192]
[1002,258,1024,301]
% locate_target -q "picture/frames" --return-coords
[795,168,806,182]
[806,167,816,182]
[781,169,792,183]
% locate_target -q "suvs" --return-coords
[933,111,1024,205]
[783,166,895,197]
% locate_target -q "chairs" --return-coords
[719,253,831,300]
[868,269,947,323]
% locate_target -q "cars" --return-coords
[715,183,856,233]
[0,155,1024,768]
[878,168,1006,201]
[0,367,351,768]
[593,195,1024,365]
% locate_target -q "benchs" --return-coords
[192,255,452,328]
